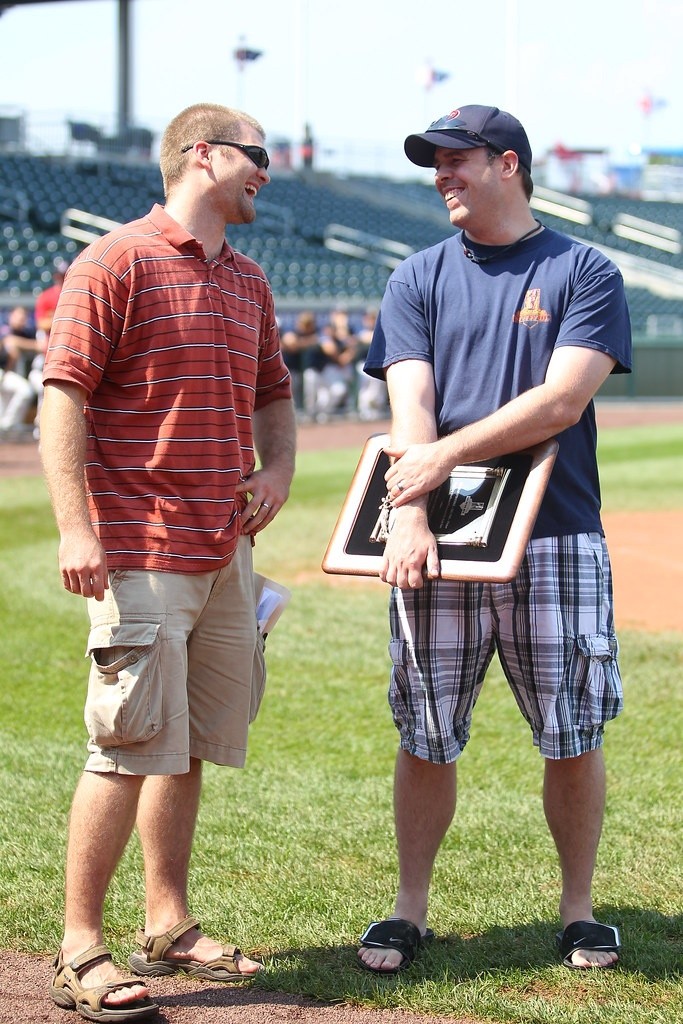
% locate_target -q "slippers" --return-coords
[555,921,620,971]
[357,913,432,975]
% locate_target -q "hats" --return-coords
[405,105,532,175]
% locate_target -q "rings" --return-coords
[397,483,406,493]
[261,503,273,510]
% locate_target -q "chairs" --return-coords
[0,151,683,343]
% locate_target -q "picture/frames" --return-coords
[320,432,561,584]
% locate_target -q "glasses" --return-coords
[427,115,506,155]
[183,142,269,171]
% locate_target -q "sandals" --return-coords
[130,919,267,981]
[53,944,161,1022]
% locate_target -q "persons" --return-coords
[32,260,75,342]
[0,306,48,438]
[344,101,638,975]
[34,104,305,1024]
[342,301,394,426]
[276,301,329,405]
[314,307,361,419]
[27,315,58,441]
